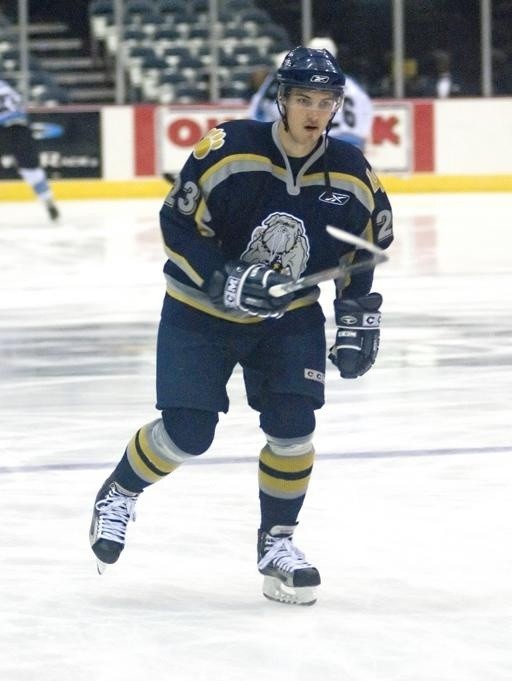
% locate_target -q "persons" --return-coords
[246,36,375,156]
[87,44,395,588]
[0,77,60,221]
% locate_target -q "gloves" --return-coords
[206,259,297,321]
[326,292,384,380]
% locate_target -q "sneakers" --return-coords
[255,520,323,589]
[89,477,144,566]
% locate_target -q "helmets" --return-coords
[274,45,347,99]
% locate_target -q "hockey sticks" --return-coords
[268,225,389,298]
